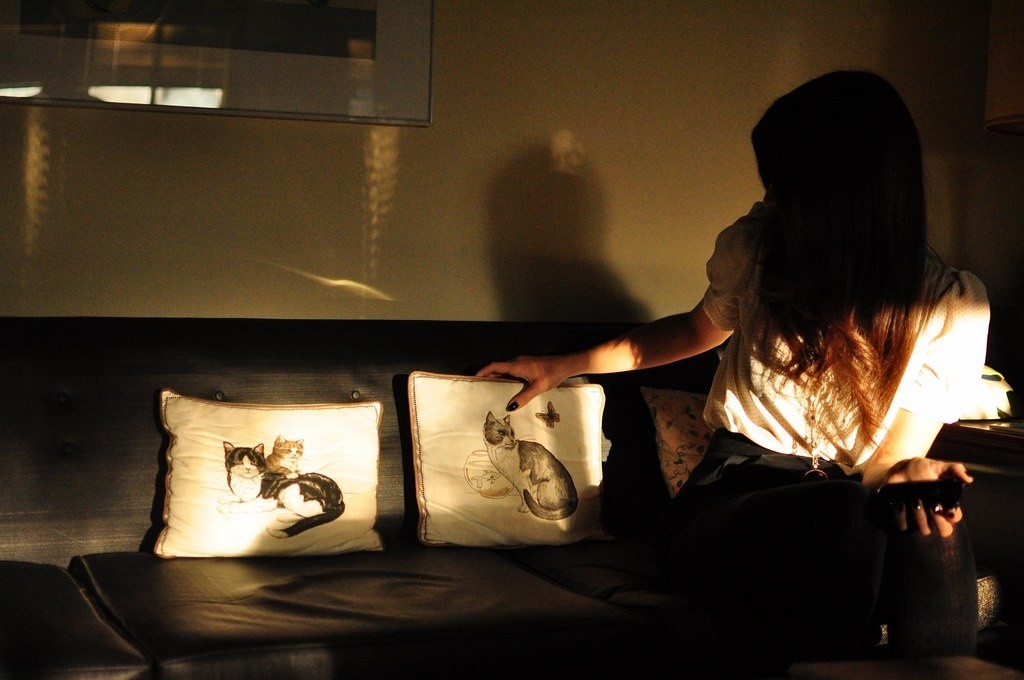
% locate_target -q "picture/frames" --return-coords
[0,0,436,127]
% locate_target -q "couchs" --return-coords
[0,315,1007,680]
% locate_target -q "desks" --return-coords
[927,418,1024,472]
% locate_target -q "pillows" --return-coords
[639,386,714,494]
[154,387,384,559]
[407,371,616,550]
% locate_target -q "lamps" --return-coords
[984,0,1024,136]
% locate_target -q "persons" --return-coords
[476,68,990,680]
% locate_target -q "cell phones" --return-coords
[877,477,965,518]
